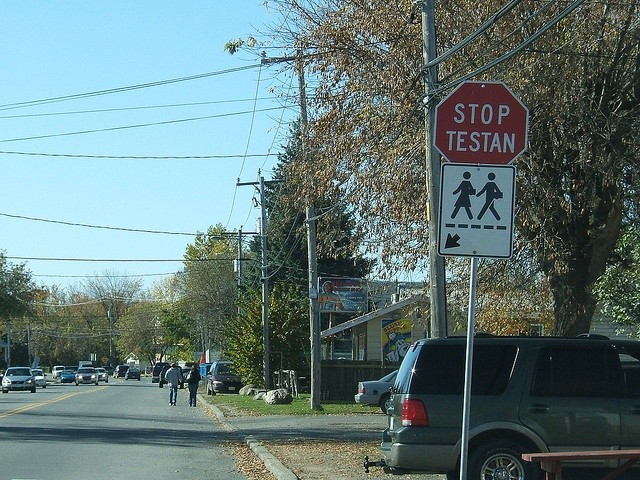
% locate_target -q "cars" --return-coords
[354,369,399,415]
[32,369,47,388]
[74,367,98,386]
[125,368,140,380]
[0,367,37,393]
[60,371,74,383]
[95,368,108,383]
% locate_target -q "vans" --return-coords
[52,366,64,379]
[380,333,639,480]
[152,363,170,383]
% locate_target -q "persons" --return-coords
[164,363,183,406]
[319,281,344,310]
[185,365,201,407]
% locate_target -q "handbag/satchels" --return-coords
[186,371,193,381]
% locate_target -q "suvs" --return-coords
[159,366,184,388]
[113,365,129,378]
[207,361,241,396]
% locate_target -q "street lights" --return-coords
[210,236,269,386]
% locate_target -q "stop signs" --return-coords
[434,81,529,165]
